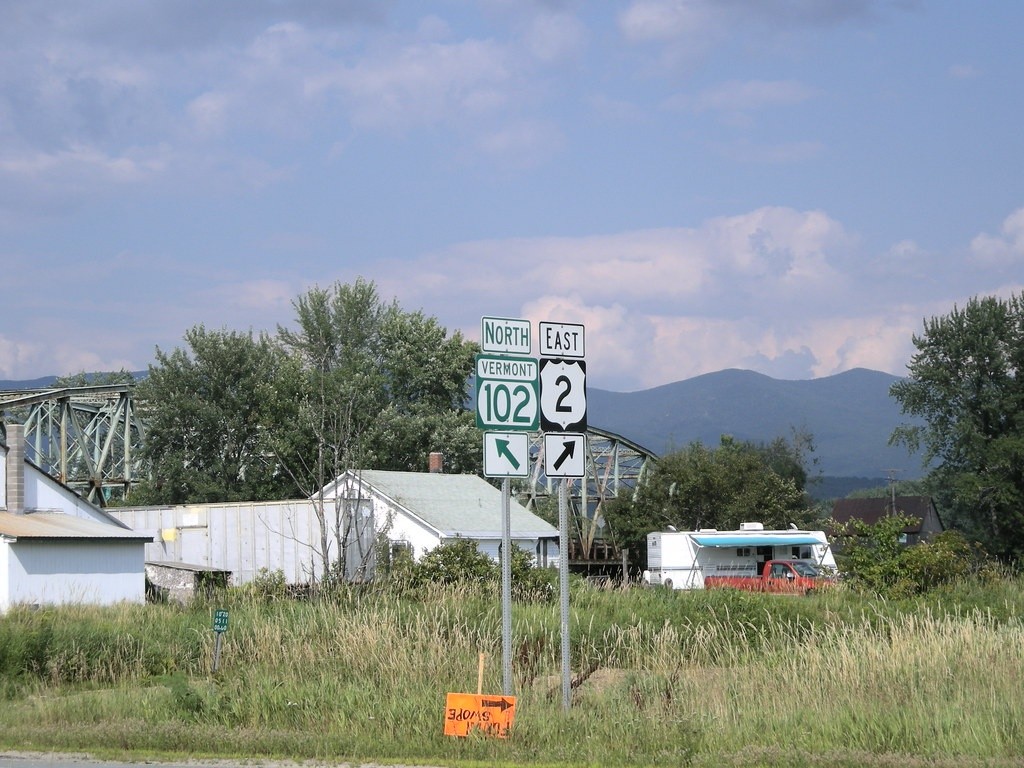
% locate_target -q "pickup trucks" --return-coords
[702,559,838,598]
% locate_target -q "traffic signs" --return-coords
[443,691,517,741]
